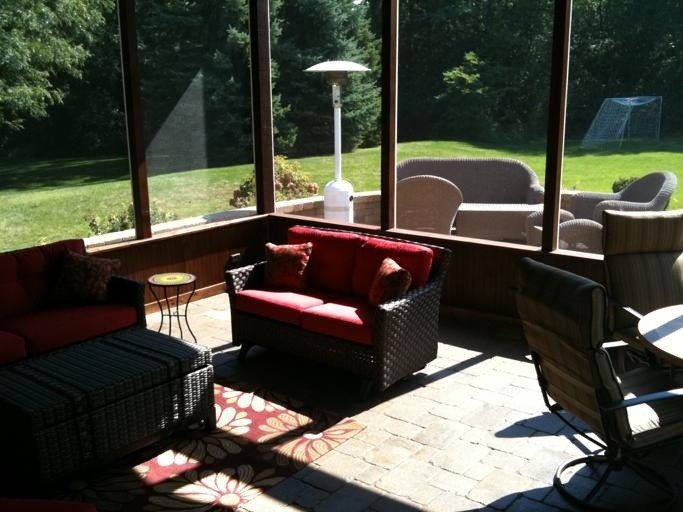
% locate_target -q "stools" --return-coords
[147,272,197,345]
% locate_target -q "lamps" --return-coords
[301,61,371,223]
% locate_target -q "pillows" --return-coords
[265,242,313,290]
[368,257,411,309]
[54,248,122,307]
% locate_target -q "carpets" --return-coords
[71,382,367,512]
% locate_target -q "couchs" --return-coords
[569,170,677,224]
[225,225,452,393]
[1,240,145,365]
[559,219,603,253]
[397,157,545,203]
[397,175,462,234]
[524,209,575,247]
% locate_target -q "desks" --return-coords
[457,203,544,240]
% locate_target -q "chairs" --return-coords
[601,211,683,371]
[514,256,683,512]
